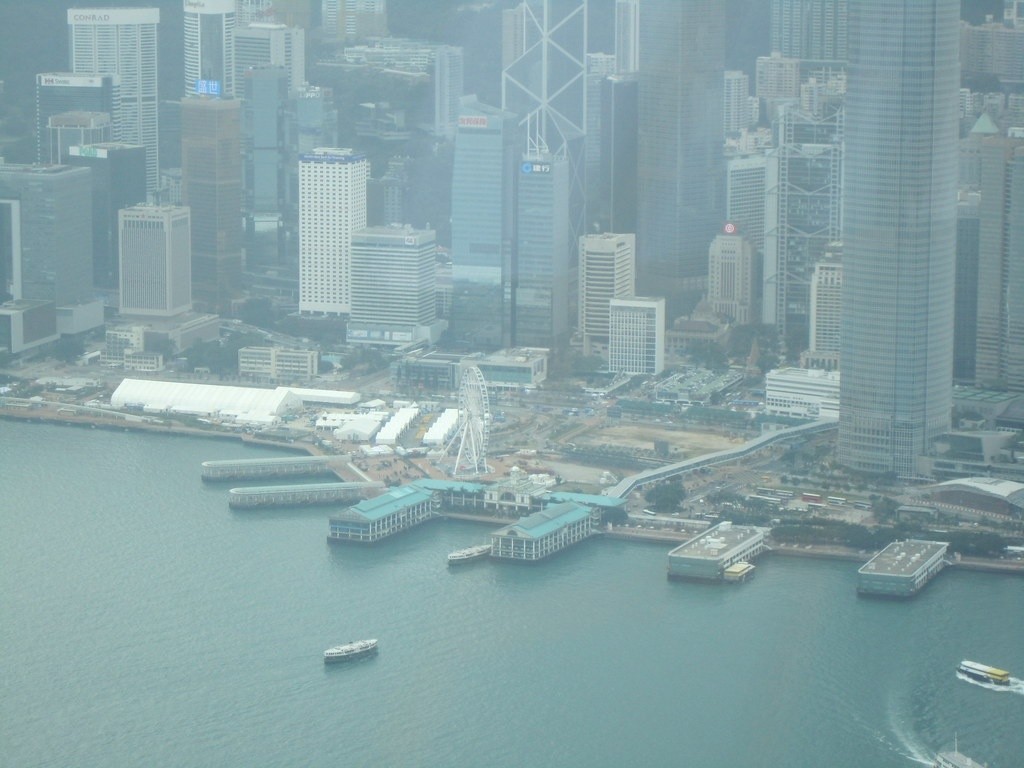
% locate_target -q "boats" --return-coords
[448,544,492,565]
[322,638,379,662]
[956,659,1010,686]
